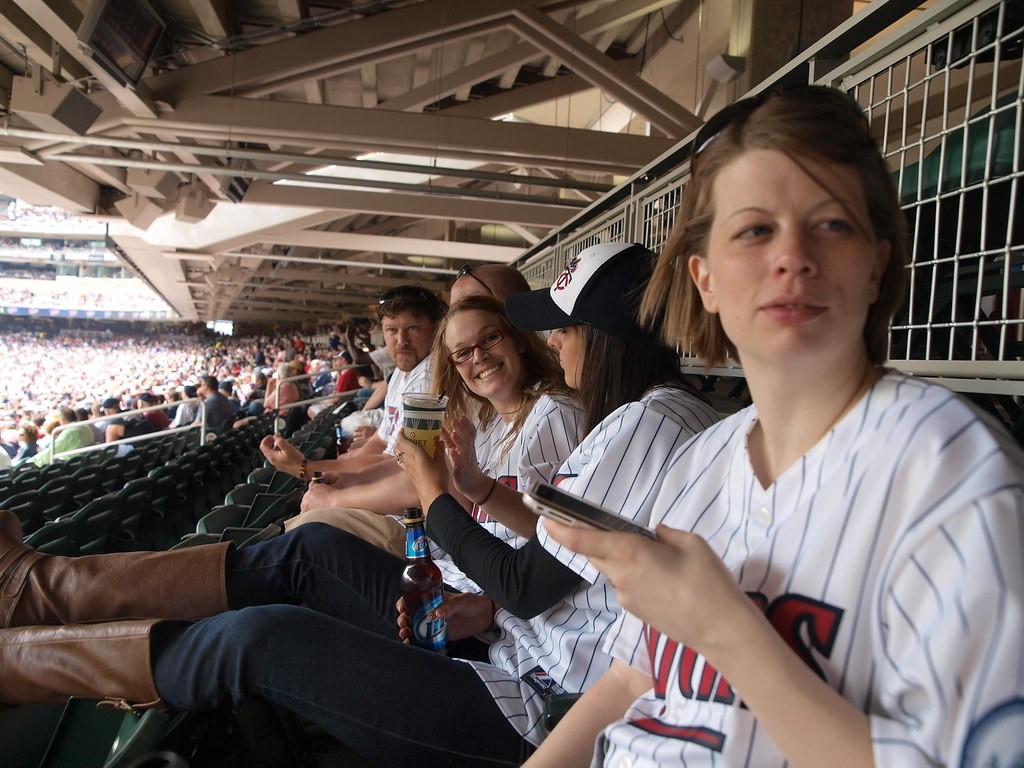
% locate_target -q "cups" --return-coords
[402,393,450,458]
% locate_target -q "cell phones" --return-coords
[522,477,661,540]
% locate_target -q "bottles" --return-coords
[401,505,451,657]
[335,424,351,454]
[312,471,326,486]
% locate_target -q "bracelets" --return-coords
[484,596,496,633]
[369,381,373,388]
[299,459,307,478]
[478,479,496,505]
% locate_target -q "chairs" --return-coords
[0,396,349,768]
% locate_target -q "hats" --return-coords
[140,393,154,402]
[332,350,353,362]
[289,360,303,371]
[185,386,196,398]
[219,382,233,392]
[100,397,119,412]
[504,242,675,346]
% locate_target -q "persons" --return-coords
[0,199,718,768]
[518,86,1024,768]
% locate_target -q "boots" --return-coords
[0,618,201,715]
[0,509,237,630]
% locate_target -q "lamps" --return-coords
[126,151,181,199]
[706,54,745,84]
[10,74,102,137]
[174,190,217,224]
[114,194,164,231]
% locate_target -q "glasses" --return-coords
[376,287,428,305]
[690,85,875,185]
[446,327,512,366]
[455,262,493,296]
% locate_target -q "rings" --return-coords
[395,452,405,461]
